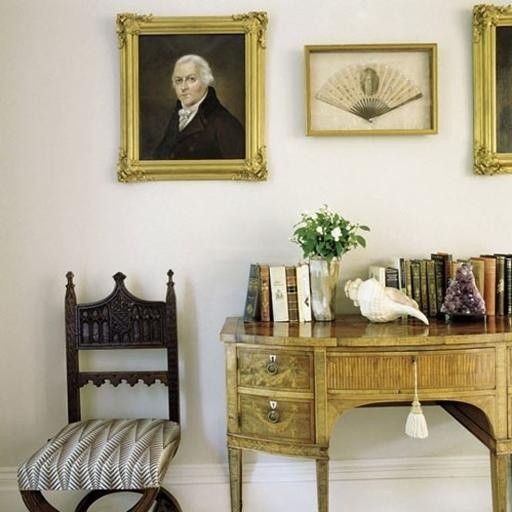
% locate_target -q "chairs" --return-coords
[16,270,183,512]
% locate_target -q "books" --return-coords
[238,248,512,324]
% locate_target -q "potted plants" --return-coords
[289,204,369,322]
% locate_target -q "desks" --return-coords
[221,316,511,512]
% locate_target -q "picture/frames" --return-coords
[303,42,438,137]
[470,4,512,177]
[116,11,267,182]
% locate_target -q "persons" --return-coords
[152,52,246,159]
